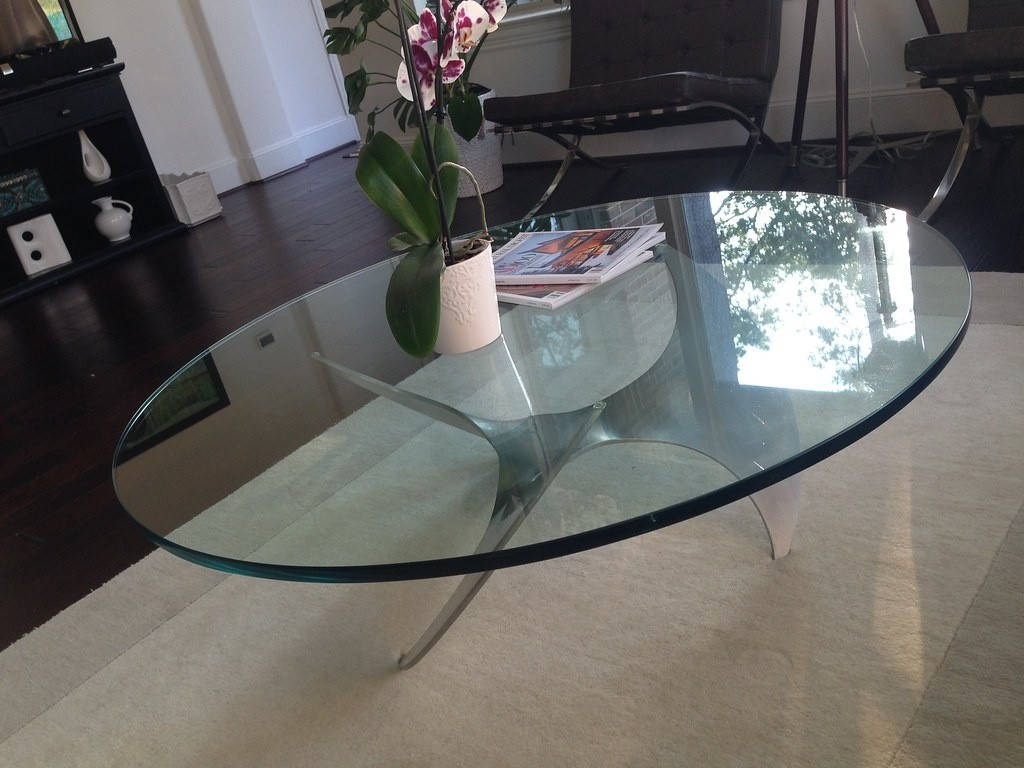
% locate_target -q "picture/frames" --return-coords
[114,349,232,468]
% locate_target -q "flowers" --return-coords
[314,0,508,146]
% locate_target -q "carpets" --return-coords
[0,259,1024,768]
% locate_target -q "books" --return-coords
[492,221,667,311]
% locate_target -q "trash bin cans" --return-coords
[427,83,503,199]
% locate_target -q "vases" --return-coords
[428,83,505,199]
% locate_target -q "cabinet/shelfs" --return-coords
[0,57,188,310]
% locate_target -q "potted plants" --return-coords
[353,0,500,355]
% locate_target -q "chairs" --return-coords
[904,0,1024,226]
[484,0,791,221]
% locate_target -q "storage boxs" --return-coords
[0,167,50,218]
[159,171,223,228]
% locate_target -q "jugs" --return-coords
[91,195,133,243]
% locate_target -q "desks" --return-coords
[111,190,975,672]
